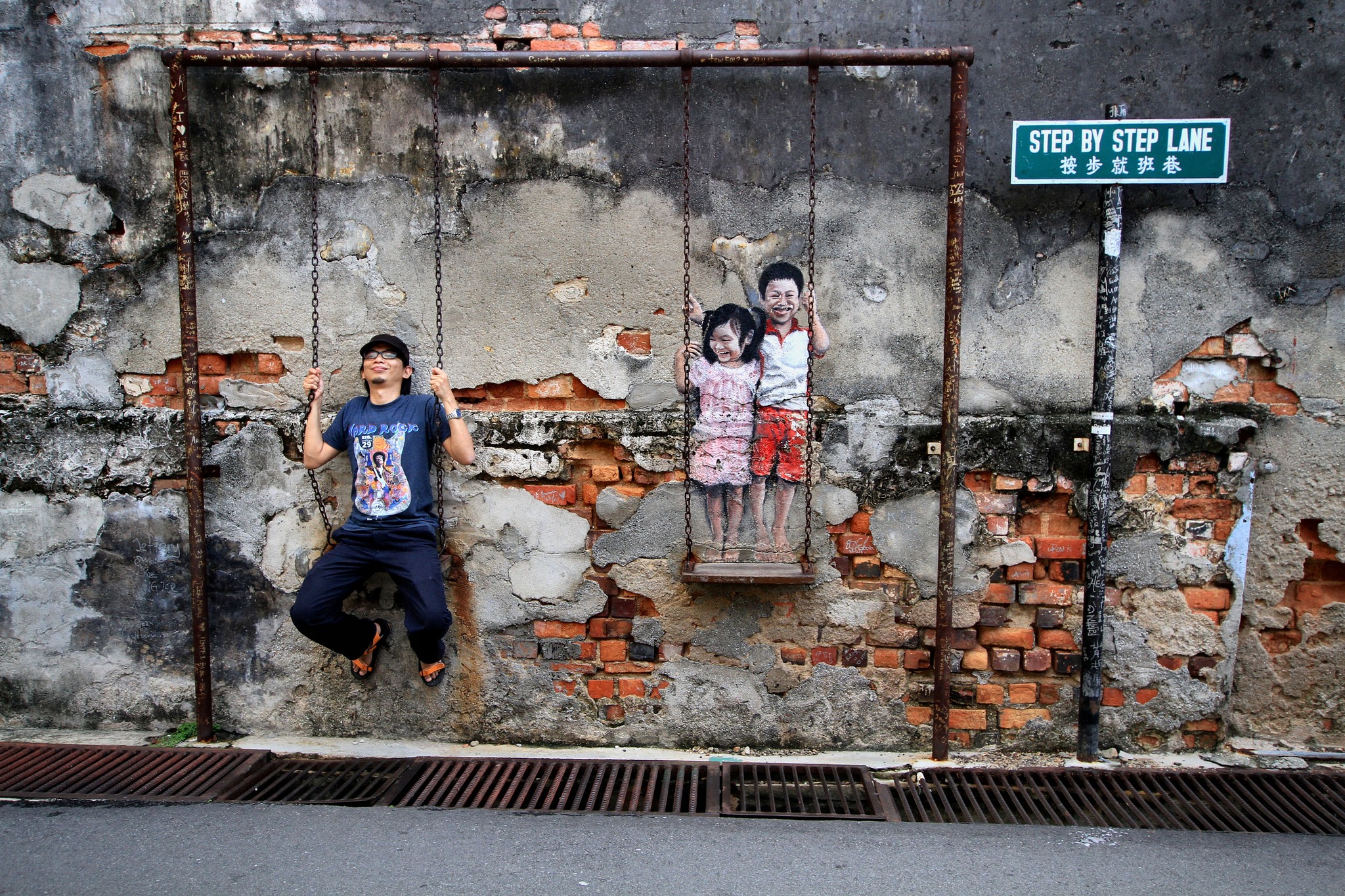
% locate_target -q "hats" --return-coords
[360,334,410,366]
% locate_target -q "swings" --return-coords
[298,67,449,608]
[678,58,821,590]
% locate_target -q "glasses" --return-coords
[363,349,399,359]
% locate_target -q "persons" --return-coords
[284,331,474,688]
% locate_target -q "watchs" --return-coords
[444,407,463,422]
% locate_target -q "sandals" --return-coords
[419,655,446,687]
[350,618,391,680]
